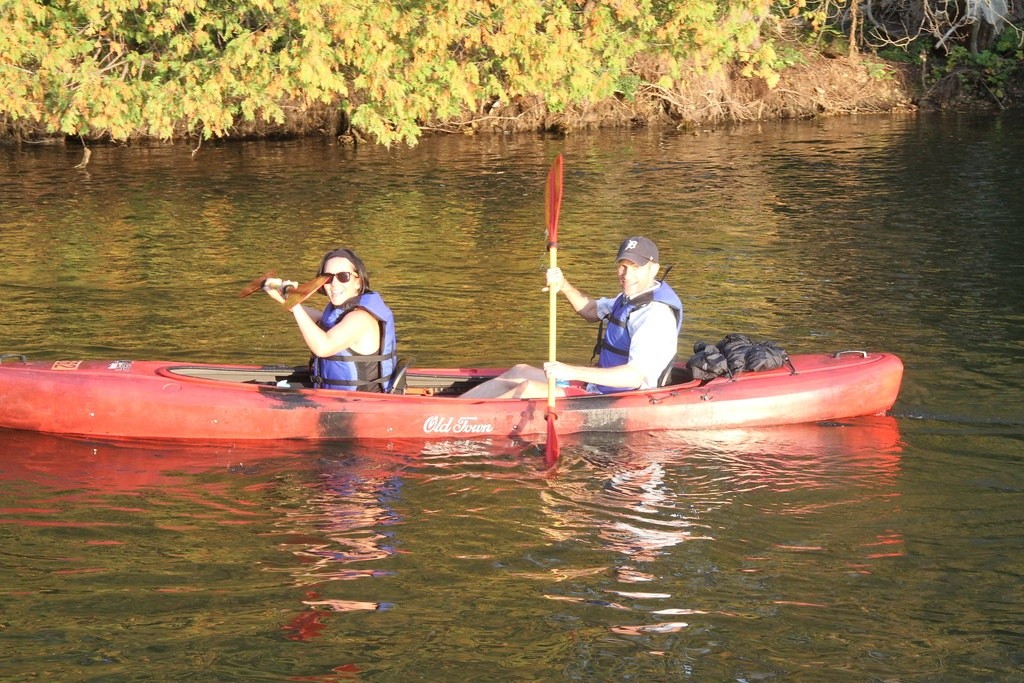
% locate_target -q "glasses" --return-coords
[321,272,358,284]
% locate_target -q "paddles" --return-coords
[544,153,564,469]
[237,272,333,313]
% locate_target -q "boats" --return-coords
[0,352,903,443]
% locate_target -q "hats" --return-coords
[614,235,659,266]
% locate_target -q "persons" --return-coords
[457,236,684,399]
[263,249,396,393]
